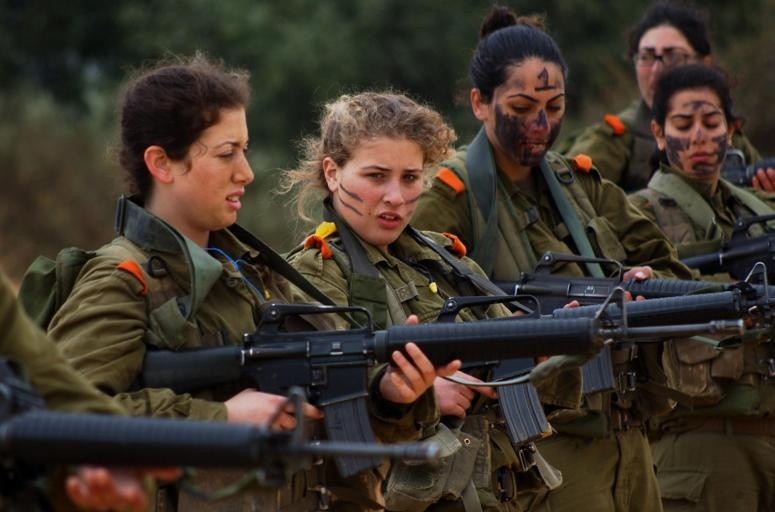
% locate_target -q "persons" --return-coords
[628,62,775,510]
[2,277,184,512]
[568,9,775,199]
[280,87,582,510]
[48,64,462,510]
[413,10,697,511]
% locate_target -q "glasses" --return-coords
[631,48,700,69]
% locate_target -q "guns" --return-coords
[140,302,746,483]
[671,210,774,282]
[0,369,442,512]
[714,148,775,191]
[429,287,775,449]
[486,250,775,321]
